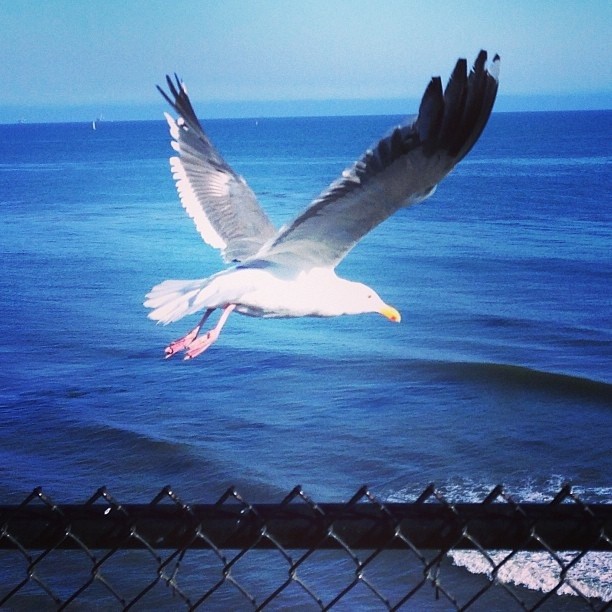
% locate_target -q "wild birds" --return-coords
[144,48,503,360]
